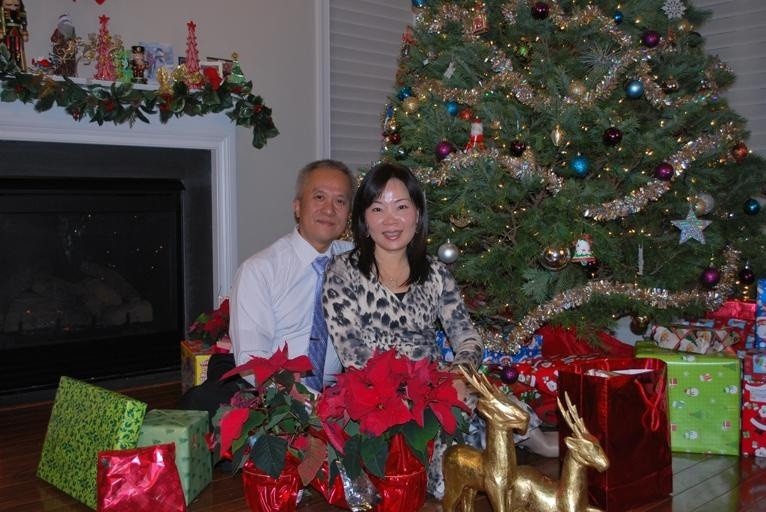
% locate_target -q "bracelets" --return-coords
[450,365,472,384]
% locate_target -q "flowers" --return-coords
[205,342,472,480]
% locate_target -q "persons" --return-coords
[320,162,559,502]
[0,1,28,73]
[126,46,150,83]
[177,157,355,475]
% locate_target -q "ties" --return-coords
[304,256,329,392]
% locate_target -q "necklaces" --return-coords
[380,274,408,286]
